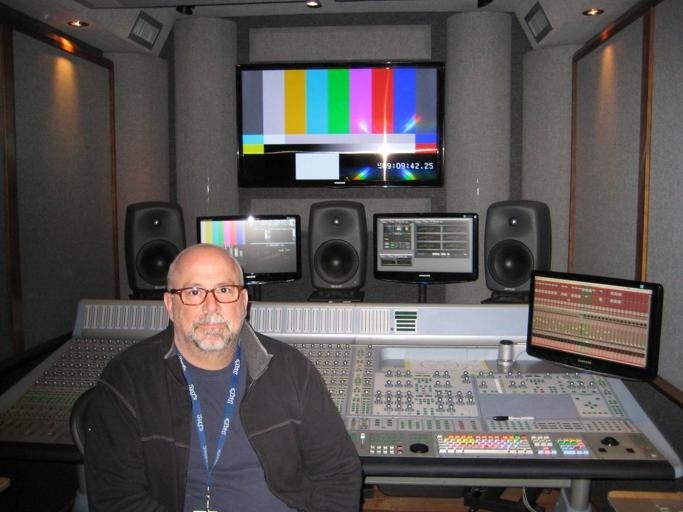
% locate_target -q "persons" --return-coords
[83,243,363,512]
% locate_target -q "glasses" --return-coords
[170,285,245,305]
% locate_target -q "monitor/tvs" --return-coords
[525,270,664,382]
[373,213,479,284]
[236,59,446,188]
[196,214,302,286]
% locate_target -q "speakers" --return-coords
[308,200,367,302]
[123,201,186,299]
[480,199,551,304]
